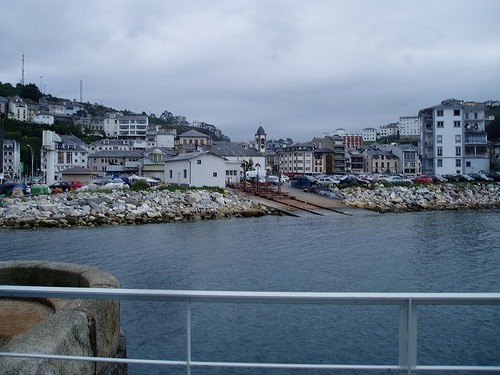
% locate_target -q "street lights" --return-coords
[26,144,34,184]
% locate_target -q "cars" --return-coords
[0,176,162,194]
[264,170,500,190]
